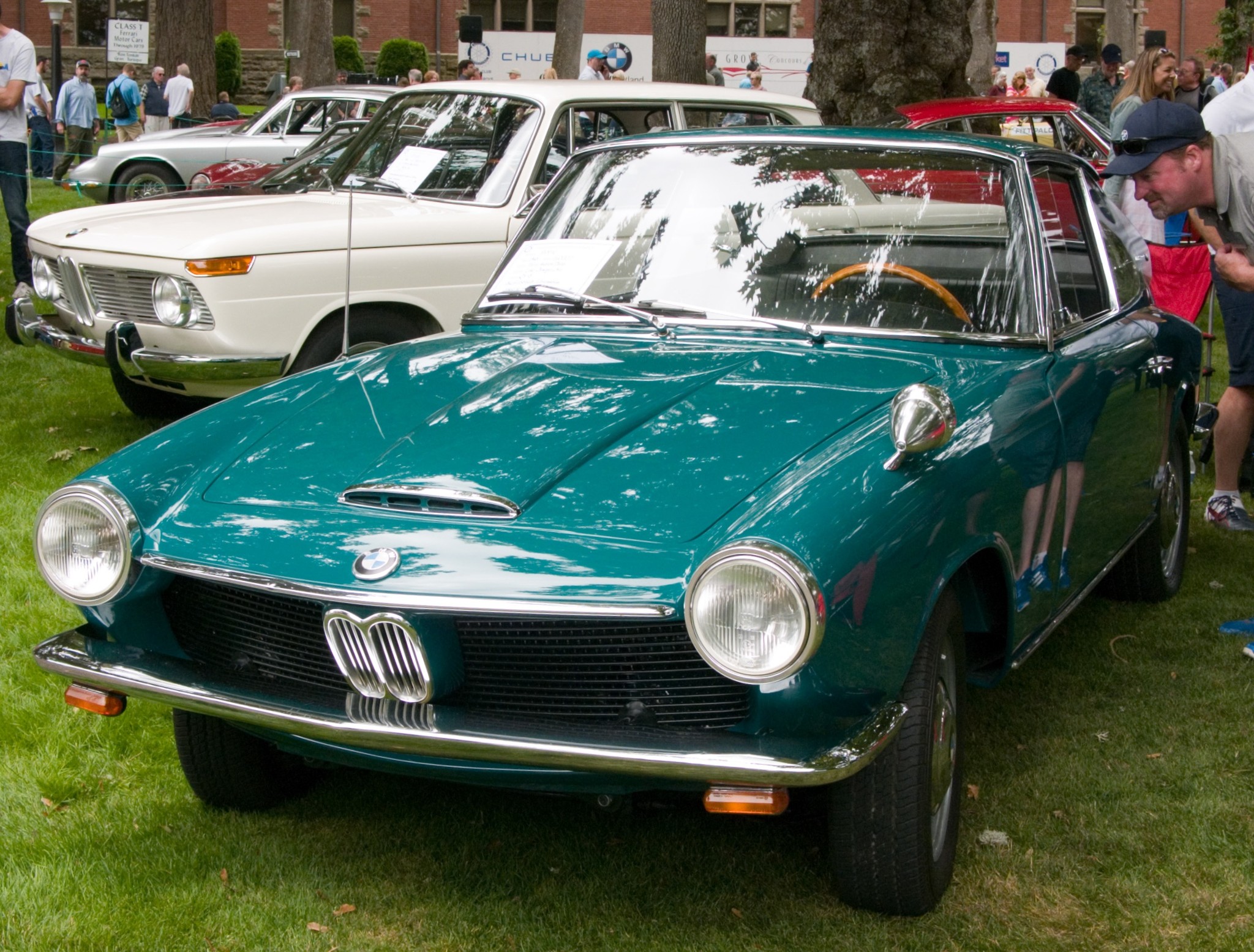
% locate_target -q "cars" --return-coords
[7,83,1198,414]
[30,126,1196,920]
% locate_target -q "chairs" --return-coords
[1164,209,1193,245]
[192,116,233,127]
[1143,240,1216,402]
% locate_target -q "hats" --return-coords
[1098,100,1205,179]
[1102,44,1123,64]
[587,49,608,59]
[1064,45,1088,58]
[507,68,521,75]
[76,60,90,68]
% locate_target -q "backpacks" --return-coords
[111,78,130,119]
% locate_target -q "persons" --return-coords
[399,48,627,143]
[1013,307,1200,617]
[163,63,194,129]
[990,43,1254,291]
[830,550,885,631]
[283,76,303,119]
[53,60,101,185]
[106,64,143,143]
[139,66,170,133]
[705,52,815,127]
[334,68,359,119]
[210,91,239,122]
[0,8,54,303]
[1186,64,1254,532]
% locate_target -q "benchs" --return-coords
[777,274,1100,317]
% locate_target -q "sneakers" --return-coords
[1243,641,1254,657]
[1015,574,1029,612]
[1029,564,1054,594]
[1058,562,1073,589]
[1205,496,1254,531]
[12,282,35,299]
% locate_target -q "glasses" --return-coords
[1112,136,1196,156]
[1176,69,1196,74]
[750,78,757,80]
[156,73,165,76]
[1153,49,1168,64]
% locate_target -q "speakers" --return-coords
[459,15,483,43]
[1144,30,1166,50]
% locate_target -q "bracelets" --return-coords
[354,107,358,111]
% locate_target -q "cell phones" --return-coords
[601,66,605,73]
